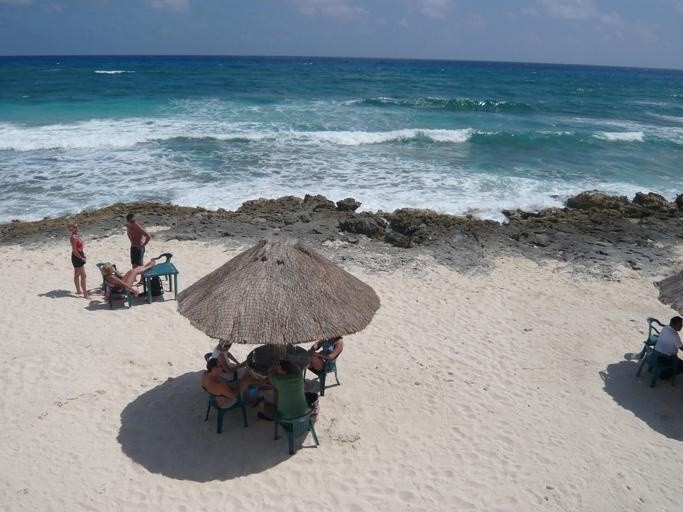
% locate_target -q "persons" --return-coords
[126,213,152,267]
[648,316,683,381]
[202,334,343,423]
[101,257,156,298]
[70,223,93,301]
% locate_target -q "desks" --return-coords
[142,263,179,304]
[247,344,311,387]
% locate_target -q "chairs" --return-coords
[96,262,133,307]
[150,253,173,292]
[635,317,676,388]
[198,349,344,455]
